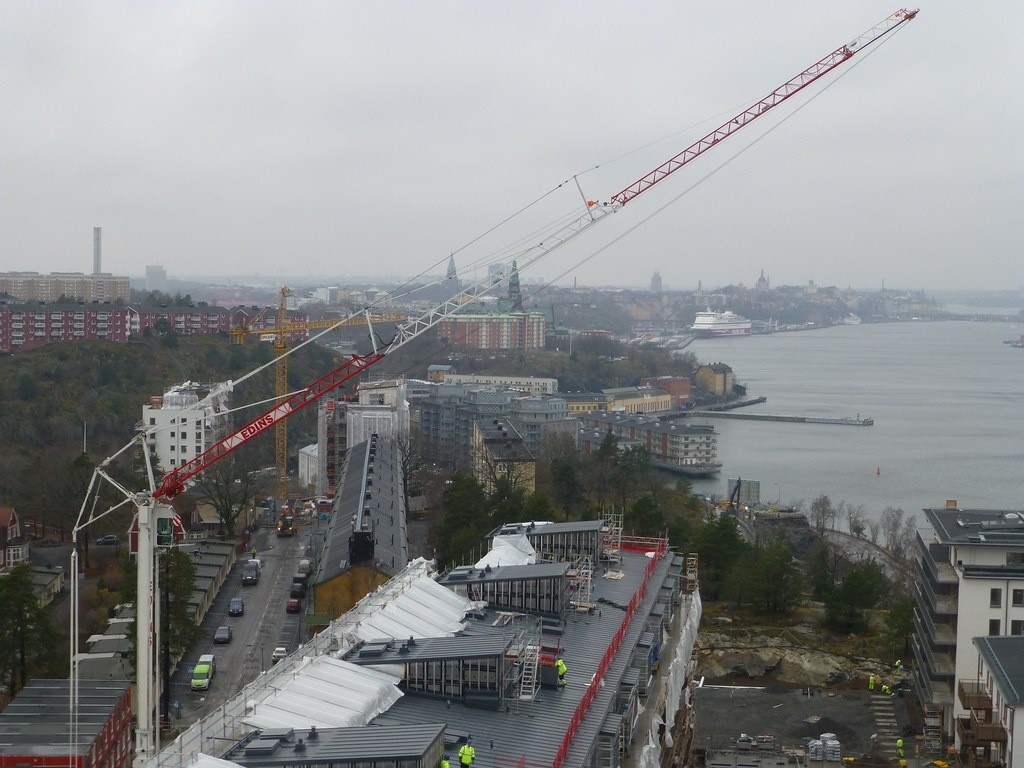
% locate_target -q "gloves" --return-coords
[472,758,474,765]
[459,757,462,762]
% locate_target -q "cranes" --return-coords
[229,284,408,500]
[69,8,920,768]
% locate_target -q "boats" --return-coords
[1004,336,1024,348]
[570,410,722,474]
[691,310,752,337]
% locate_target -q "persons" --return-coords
[895,737,904,758]
[294,525,298,534]
[284,509,288,516]
[279,519,283,530]
[553,658,567,685]
[869,674,875,690]
[291,508,295,515]
[252,547,256,559]
[894,660,901,669]
[880,683,891,694]
[459,739,475,768]
[440,754,450,768]
[897,756,907,768]
[274,516,275,521]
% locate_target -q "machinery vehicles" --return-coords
[277,506,298,537]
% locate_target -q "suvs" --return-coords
[230,598,244,616]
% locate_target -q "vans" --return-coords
[298,560,310,574]
[198,655,216,677]
[293,574,308,591]
[191,665,211,690]
[291,583,305,597]
[241,562,259,586]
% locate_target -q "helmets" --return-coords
[445,754,449,760]
[553,657,557,660]
[467,739,472,744]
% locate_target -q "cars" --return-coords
[286,600,301,613]
[96,535,119,545]
[214,626,232,644]
[272,647,288,662]
